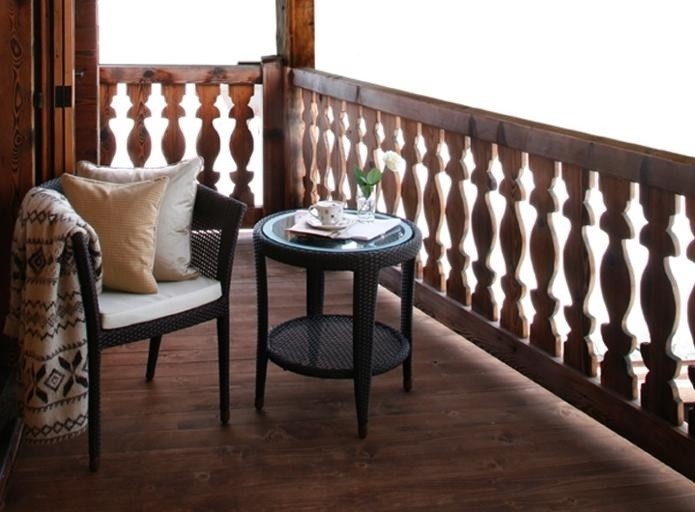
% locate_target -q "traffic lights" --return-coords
[59,173,169,294]
[75,155,206,281]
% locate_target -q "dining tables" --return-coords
[355,167,383,221]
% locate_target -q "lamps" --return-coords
[29,171,247,472]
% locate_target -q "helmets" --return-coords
[307,213,351,229]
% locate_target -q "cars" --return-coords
[355,186,377,223]
[308,201,343,225]
